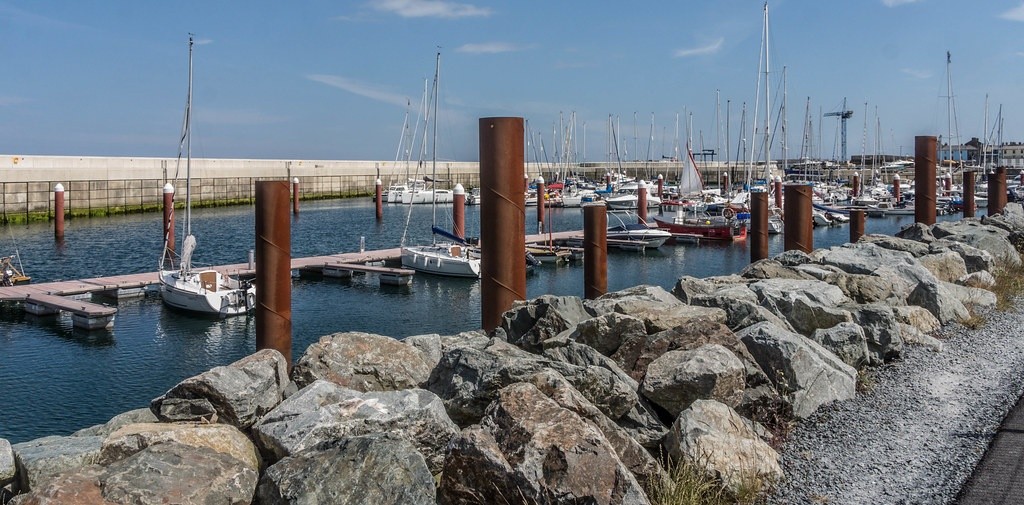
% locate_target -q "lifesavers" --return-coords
[12,157,18,163]
[723,209,733,219]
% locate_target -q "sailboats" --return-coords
[156,31,257,316]
[369,1,1024,278]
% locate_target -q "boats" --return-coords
[0,203,31,287]
